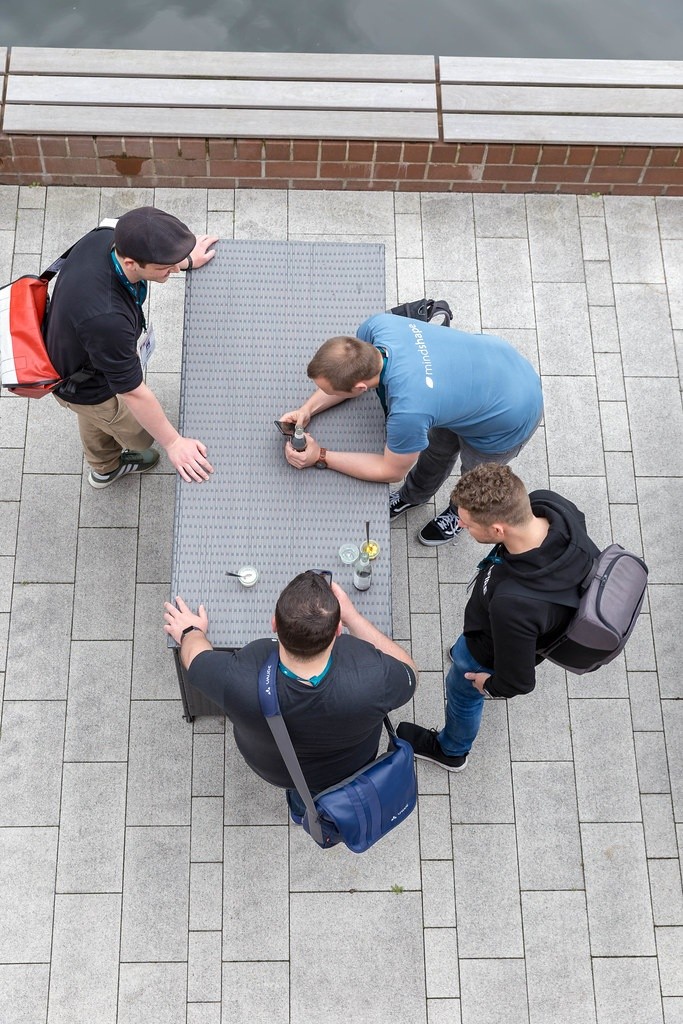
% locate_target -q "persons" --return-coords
[280,312,543,546]
[44,207,218,488]
[163,568,419,823]
[146,342,151,349]
[395,463,600,773]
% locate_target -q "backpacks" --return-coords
[493,536,648,676]
[390,298,453,328]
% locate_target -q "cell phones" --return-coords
[274,420,308,436]
[312,568,332,586]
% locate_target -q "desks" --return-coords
[166,240,394,722]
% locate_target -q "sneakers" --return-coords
[448,645,455,662]
[395,721,470,772]
[87,447,160,489]
[389,489,425,522]
[417,505,467,547]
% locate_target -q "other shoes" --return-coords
[290,810,304,825]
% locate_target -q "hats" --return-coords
[113,206,196,265]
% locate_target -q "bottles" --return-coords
[291,424,308,452]
[354,552,373,591]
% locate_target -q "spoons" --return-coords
[224,571,252,579]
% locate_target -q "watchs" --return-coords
[180,626,203,644]
[315,448,327,469]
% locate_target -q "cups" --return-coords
[338,544,360,565]
[238,565,259,587]
[360,539,380,560]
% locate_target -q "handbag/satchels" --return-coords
[302,738,417,854]
[0,273,65,400]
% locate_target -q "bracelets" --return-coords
[181,255,193,270]
